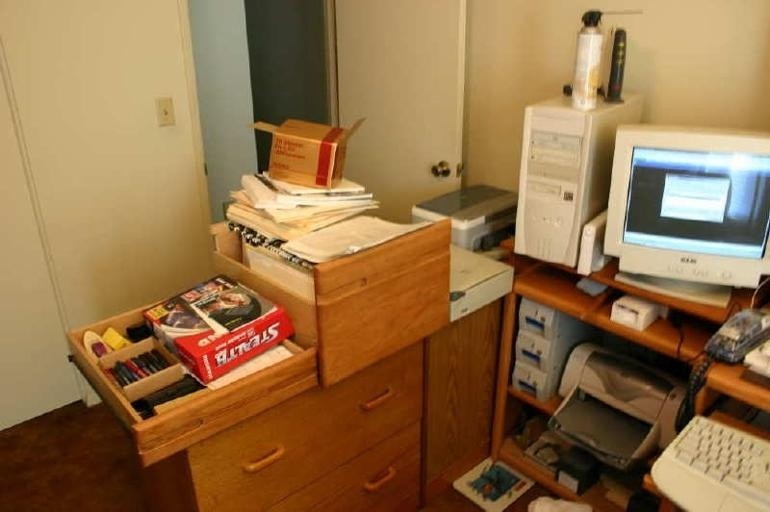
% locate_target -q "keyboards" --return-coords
[650,414,770,512]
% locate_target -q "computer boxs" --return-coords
[514,89,645,267]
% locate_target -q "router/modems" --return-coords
[576,209,612,277]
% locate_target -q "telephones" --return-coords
[703,308,770,364]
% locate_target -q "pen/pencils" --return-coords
[108,349,171,388]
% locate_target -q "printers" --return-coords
[546,342,691,473]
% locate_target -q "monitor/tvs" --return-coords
[603,124,770,308]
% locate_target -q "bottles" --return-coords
[571,11,603,110]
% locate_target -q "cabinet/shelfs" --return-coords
[422,295,501,497]
[134,337,423,511]
[500,236,770,510]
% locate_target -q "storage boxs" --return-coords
[450,246,516,322]
[247,116,371,189]
[206,219,452,384]
[67,268,314,469]
